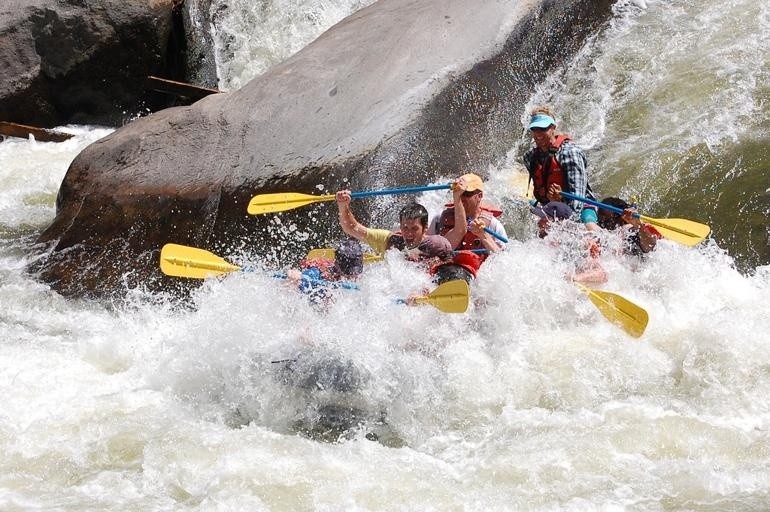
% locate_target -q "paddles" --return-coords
[389,279,469,313]
[478,224,649,339]
[555,189,710,248]
[248,182,456,214]
[160,242,359,292]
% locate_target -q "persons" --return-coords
[432,173,510,257]
[521,104,601,232]
[280,238,364,299]
[408,232,475,300]
[527,200,608,288]
[597,194,664,255]
[333,187,432,263]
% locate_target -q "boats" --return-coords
[243,332,387,441]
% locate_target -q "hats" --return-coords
[527,112,557,131]
[335,240,364,277]
[449,173,484,195]
